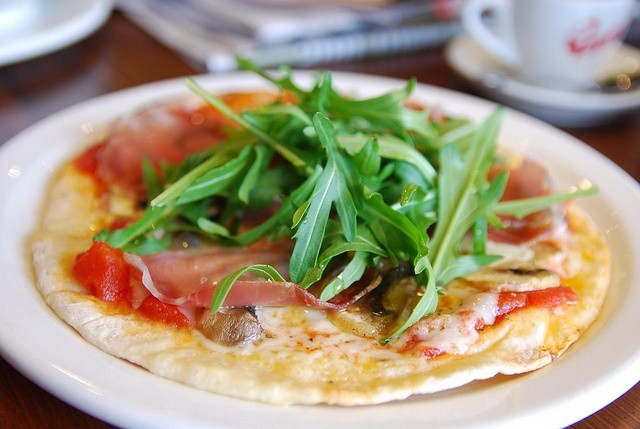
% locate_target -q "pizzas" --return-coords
[32,53,612,406]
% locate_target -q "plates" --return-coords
[2,69,640,429]
[440,24,640,132]
[0,1,117,69]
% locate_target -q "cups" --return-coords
[461,1,633,91]
